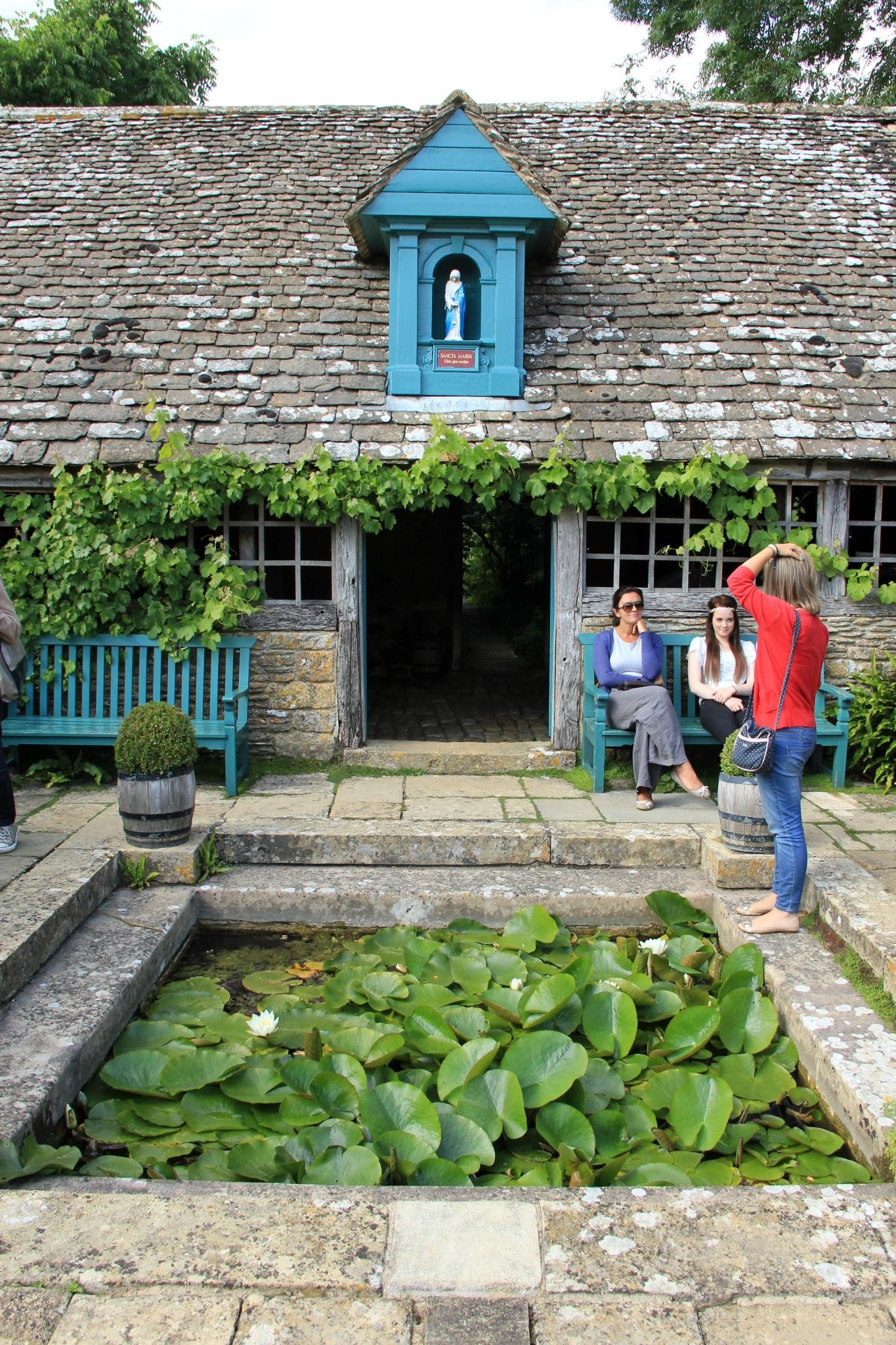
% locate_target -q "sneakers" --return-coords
[0,824,19,853]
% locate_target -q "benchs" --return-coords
[0,635,258,797]
[576,632,854,792]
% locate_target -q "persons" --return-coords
[727,543,829,935]
[0,585,26,853]
[687,596,757,745]
[593,586,711,812]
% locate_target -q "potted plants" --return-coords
[718,728,775,853]
[114,701,200,849]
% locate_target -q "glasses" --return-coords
[616,601,644,613]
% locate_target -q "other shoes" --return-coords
[636,793,654,810]
[736,903,773,915]
[670,769,710,799]
[737,916,799,933]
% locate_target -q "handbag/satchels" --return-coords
[732,720,775,774]
[616,681,662,690]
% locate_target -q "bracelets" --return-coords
[768,544,778,558]
[731,685,737,695]
